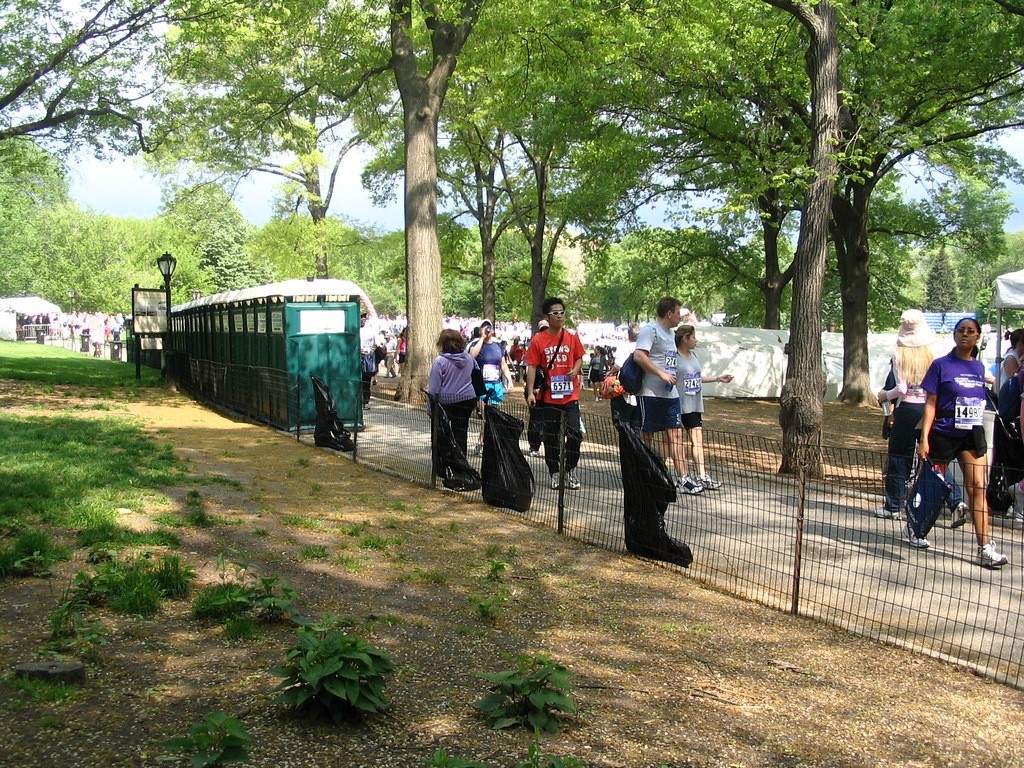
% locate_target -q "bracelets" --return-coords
[717,376,720,383]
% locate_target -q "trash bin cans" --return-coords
[36,329,45,344]
[109,342,121,361]
[79,334,91,352]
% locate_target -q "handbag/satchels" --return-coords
[533,367,547,391]
[905,460,951,539]
[471,359,487,396]
[619,353,646,396]
[361,344,385,375]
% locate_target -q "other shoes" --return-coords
[473,446,483,457]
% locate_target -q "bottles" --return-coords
[485,326,491,333]
[665,374,675,393]
[880,389,893,417]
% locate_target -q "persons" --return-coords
[361,313,381,410]
[16,311,126,356]
[874,309,1024,519]
[527,298,586,489]
[588,346,608,403]
[467,319,513,456]
[659,322,734,490]
[634,296,705,494]
[875,310,970,529]
[903,318,1008,567]
[428,329,484,458]
[360,312,690,466]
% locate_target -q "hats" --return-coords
[361,311,367,316]
[479,319,492,327]
[538,320,549,330]
[898,309,935,347]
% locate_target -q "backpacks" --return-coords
[998,363,1024,422]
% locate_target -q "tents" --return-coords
[0,297,63,341]
[992,268,1024,407]
[664,324,1016,401]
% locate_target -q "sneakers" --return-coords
[551,472,559,490]
[1008,483,1024,515]
[1006,506,1024,520]
[902,522,930,548]
[363,404,370,409]
[675,474,705,494]
[951,502,970,529]
[874,508,903,520]
[695,475,722,489]
[565,471,580,488]
[530,450,540,457]
[976,540,1008,566]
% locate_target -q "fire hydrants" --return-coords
[94,342,102,357]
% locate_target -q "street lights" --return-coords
[69,289,75,340]
[156,252,179,389]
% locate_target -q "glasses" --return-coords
[954,328,980,338]
[547,311,565,316]
